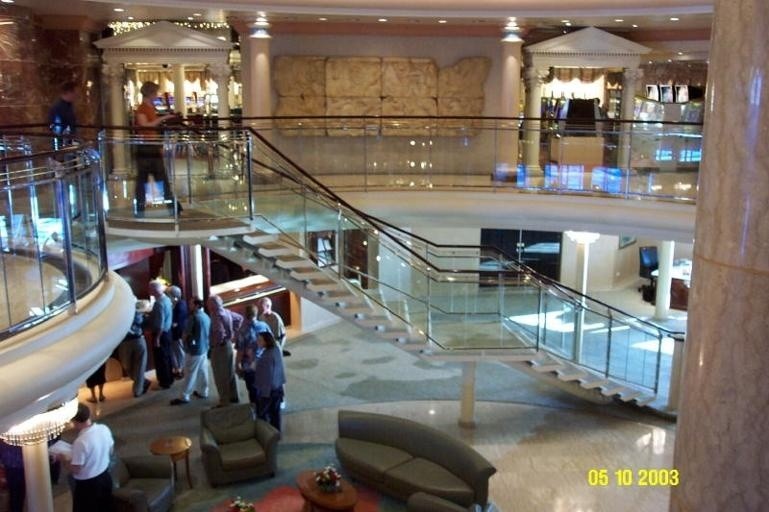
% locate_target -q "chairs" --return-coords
[639,246,658,293]
[199,403,282,488]
[108,453,175,512]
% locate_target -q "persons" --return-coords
[317,231,333,269]
[118,278,286,437]
[49,432,62,486]
[48,83,80,220]
[131,80,183,217]
[85,362,105,403]
[59,404,115,512]
[0,440,26,512]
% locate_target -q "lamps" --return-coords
[0,394,79,446]
[501,33,525,44]
[248,28,272,39]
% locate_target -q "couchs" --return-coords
[335,410,497,512]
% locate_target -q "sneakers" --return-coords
[169,397,192,405]
[193,390,209,399]
[87,393,106,403]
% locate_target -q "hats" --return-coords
[141,82,158,97]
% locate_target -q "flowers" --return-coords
[315,466,341,486]
[228,496,255,512]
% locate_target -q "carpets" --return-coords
[210,483,381,512]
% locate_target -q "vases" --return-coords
[320,484,334,492]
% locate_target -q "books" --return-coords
[48,440,72,457]
